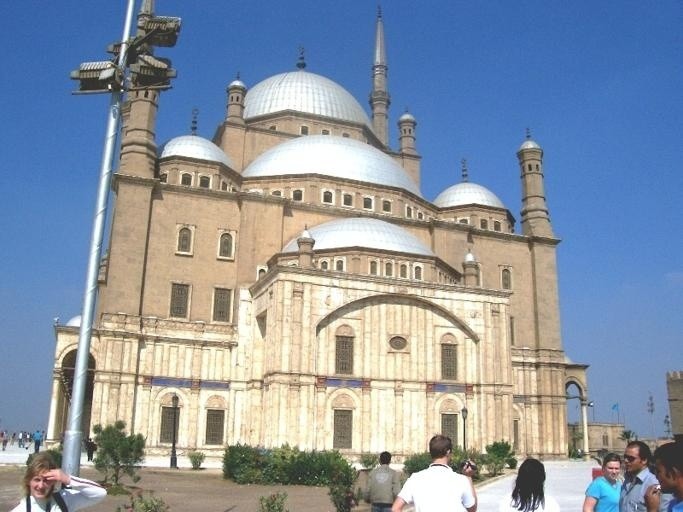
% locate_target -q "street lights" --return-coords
[170,395,179,468]
[461,408,468,450]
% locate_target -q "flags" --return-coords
[612,403,618,410]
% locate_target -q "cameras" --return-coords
[464,461,469,468]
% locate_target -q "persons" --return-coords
[0,428,47,455]
[364,450,401,512]
[8,454,106,511]
[390,434,478,512]
[581,452,622,512]
[644,440,682,511]
[618,441,660,511]
[498,458,558,511]
[85,437,95,461]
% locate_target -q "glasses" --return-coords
[624,456,639,461]
